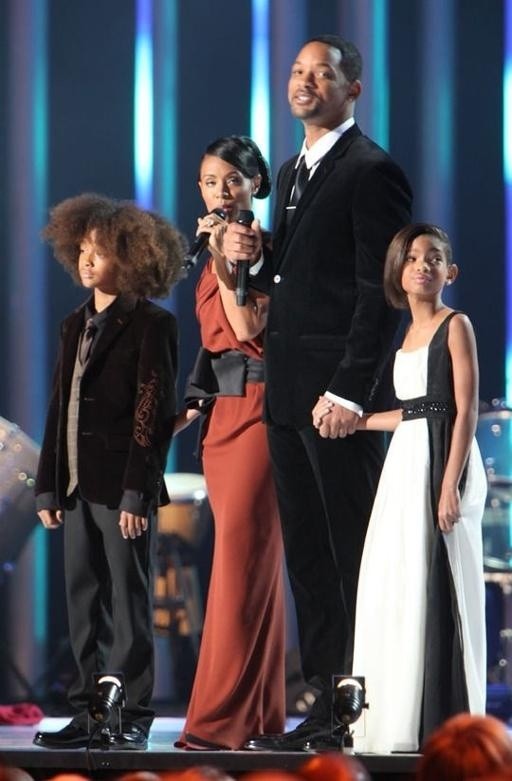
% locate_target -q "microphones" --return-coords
[233,209,254,307]
[182,208,226,272]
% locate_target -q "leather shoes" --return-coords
[33,718,103,748]
[303,721,353,752]
[244,715,332,752]
[107,723,148,751]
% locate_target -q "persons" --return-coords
[173,134,287,750]
[224,34,412,756]
[32,192,190,750]
[311,223,489,755]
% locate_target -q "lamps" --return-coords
[85,670,128,748]
[331,676,369,748]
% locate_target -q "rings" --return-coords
[208,219,214,227]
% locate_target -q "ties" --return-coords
[80,317,94,364]
[281,154,312,244]
